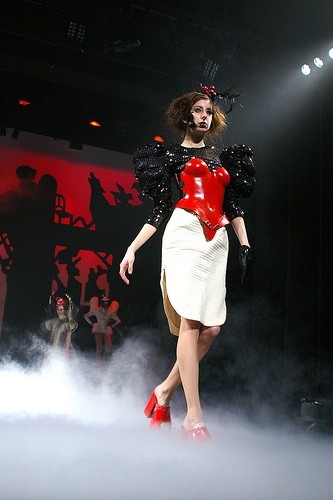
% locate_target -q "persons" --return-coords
[119,83,252,444]
[35,293,80,356]
[83,297,121,366]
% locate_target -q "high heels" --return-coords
[179,424,213,443]
[144,392,171,431]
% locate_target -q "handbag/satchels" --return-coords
[240,245,252,283]
